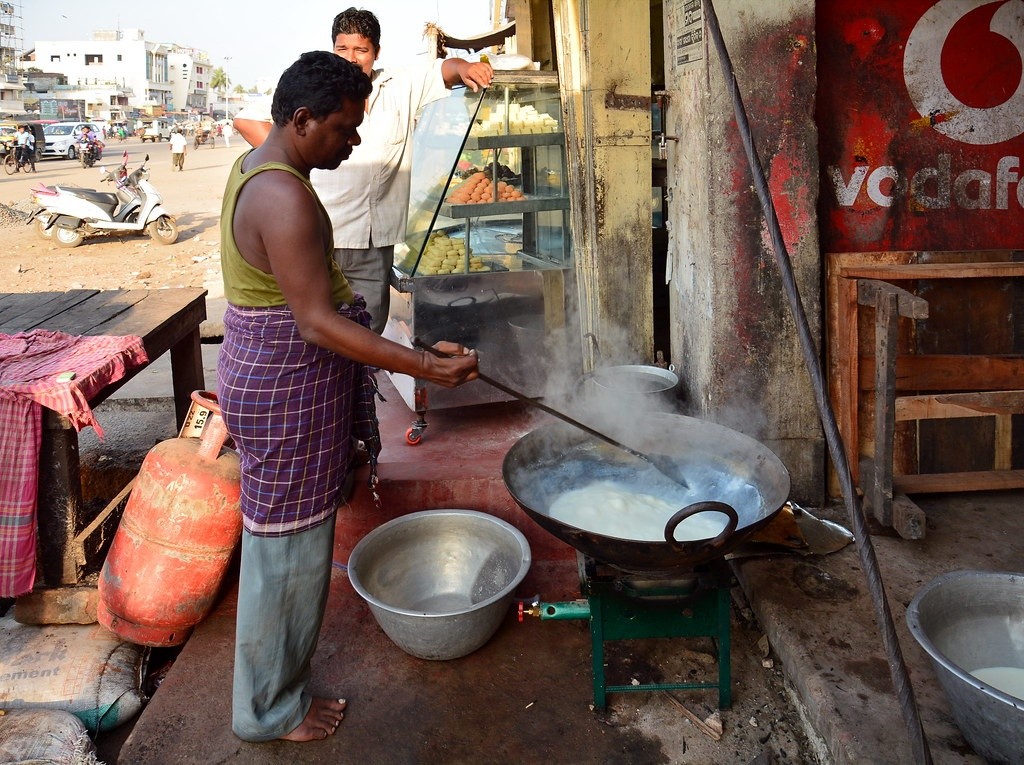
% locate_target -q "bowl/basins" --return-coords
[906,568,1024,765]
[507,316,544,357]
[345,509,532,661]
[591,365,680,415]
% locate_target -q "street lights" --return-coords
[223,56,233,122]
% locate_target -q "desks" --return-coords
[823,247,1024,542]
[1,286,208,590]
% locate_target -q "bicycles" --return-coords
[4,145,32,175]
[117,134,127,143]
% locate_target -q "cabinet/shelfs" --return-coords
[380,71,578,445]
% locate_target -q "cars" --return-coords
[30,121,104,160]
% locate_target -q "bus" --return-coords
[137,117,171,143]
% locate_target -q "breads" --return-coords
[450,104,559,137]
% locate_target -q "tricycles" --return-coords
[193,131,215,150]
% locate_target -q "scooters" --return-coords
[26,149,179,248]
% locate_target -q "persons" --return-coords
[216,52,480,744]
[223,123,233,147]
[232,6,495,337]
[0,118,234,173]
[169,128,187,171]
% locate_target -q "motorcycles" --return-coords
[0,120,46,164]
[78,141,96,168]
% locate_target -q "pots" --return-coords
[416,291,492,324]
[502,370,791,575]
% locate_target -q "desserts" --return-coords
[426,160,528,205]
[395,230,492,276]
